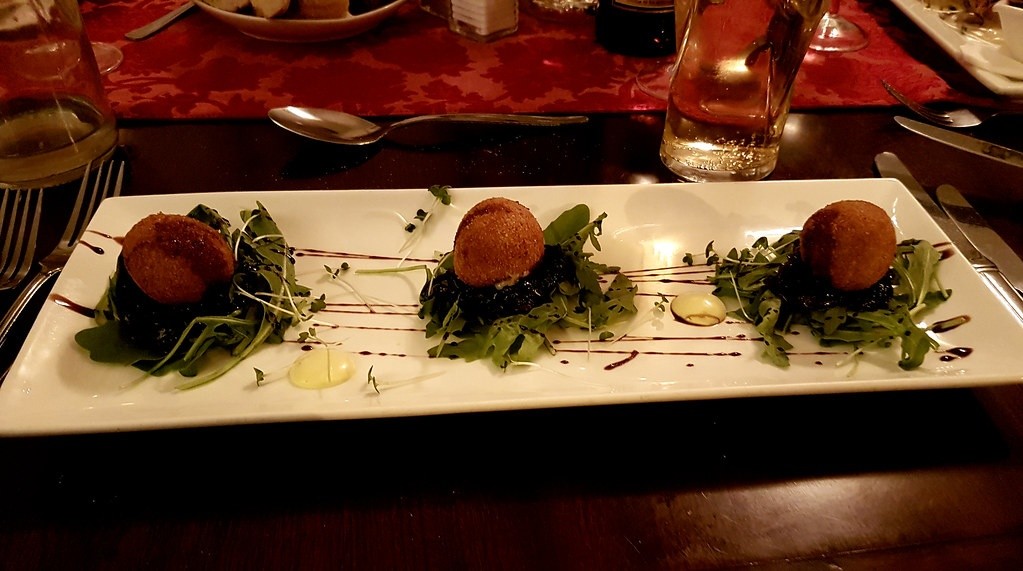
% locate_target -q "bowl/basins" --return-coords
[991,2,1022,66]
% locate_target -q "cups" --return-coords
[655,0,832,184]
[0,0,119,191]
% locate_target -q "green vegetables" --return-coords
[74,185,964,395]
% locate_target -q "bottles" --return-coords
[445,0,519,43]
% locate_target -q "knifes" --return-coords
[935,182,1023,300]
[892,115,1022,169]
[873,149,1022,324]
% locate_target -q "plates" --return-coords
[890,0,1023,99]
[2,176,1023,441]
[191,0,405,43]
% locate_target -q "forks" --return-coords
[0,184,44,295]
[877,77,1022,129]
[0,159,126,349]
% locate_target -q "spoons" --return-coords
[267,104,590,148]
